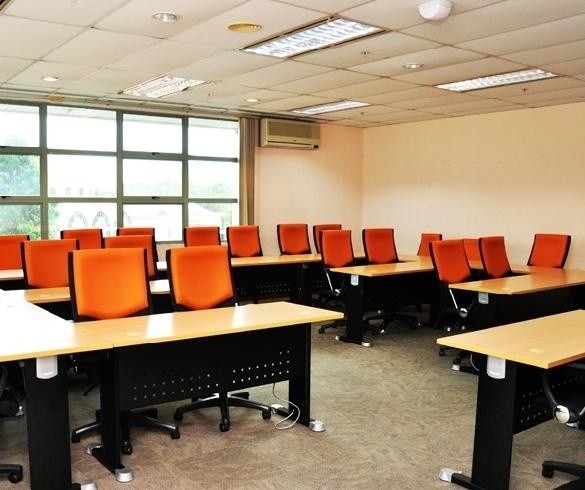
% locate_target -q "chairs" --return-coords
[538,361,585,490]
[358,225,585,373]
[0,224,355,369]
[65,248,183,453]
[164,244,272,432]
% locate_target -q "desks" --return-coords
[70,299,349,485]
[423,307,585,490]
[0,289,116,490]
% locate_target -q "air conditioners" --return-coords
[260,115,321,152]
